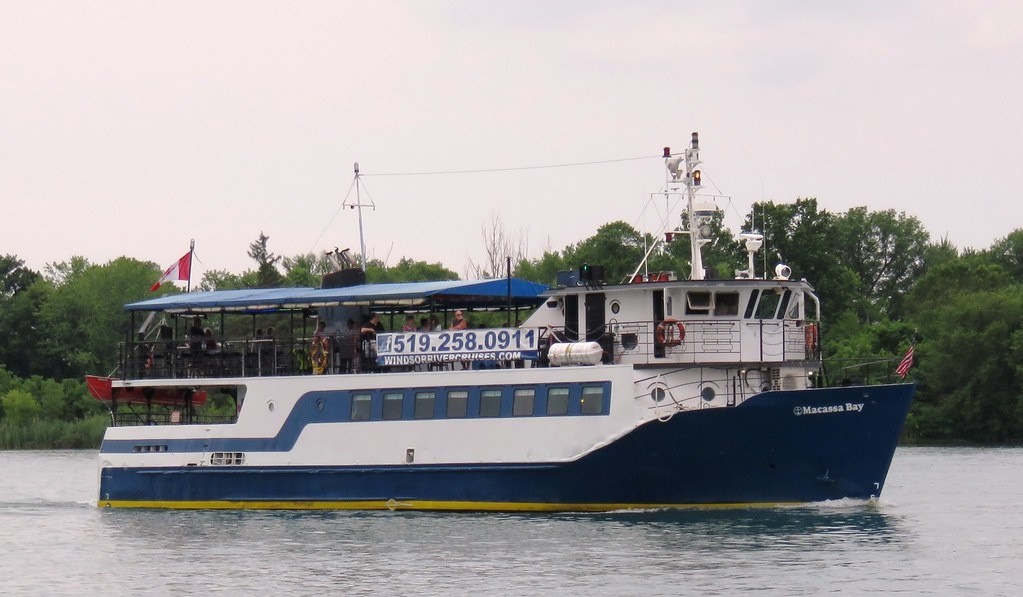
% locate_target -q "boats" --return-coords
[84,130,916,512]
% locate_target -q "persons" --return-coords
[315,310,470,374]
[204,327,218,348]
[189,317,204,351]
[250,328,275,352]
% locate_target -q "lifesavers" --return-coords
[310,336,329,373]
[655,319,685,347]
[805,322,818,352]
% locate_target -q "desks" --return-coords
[152,343,304,380]
